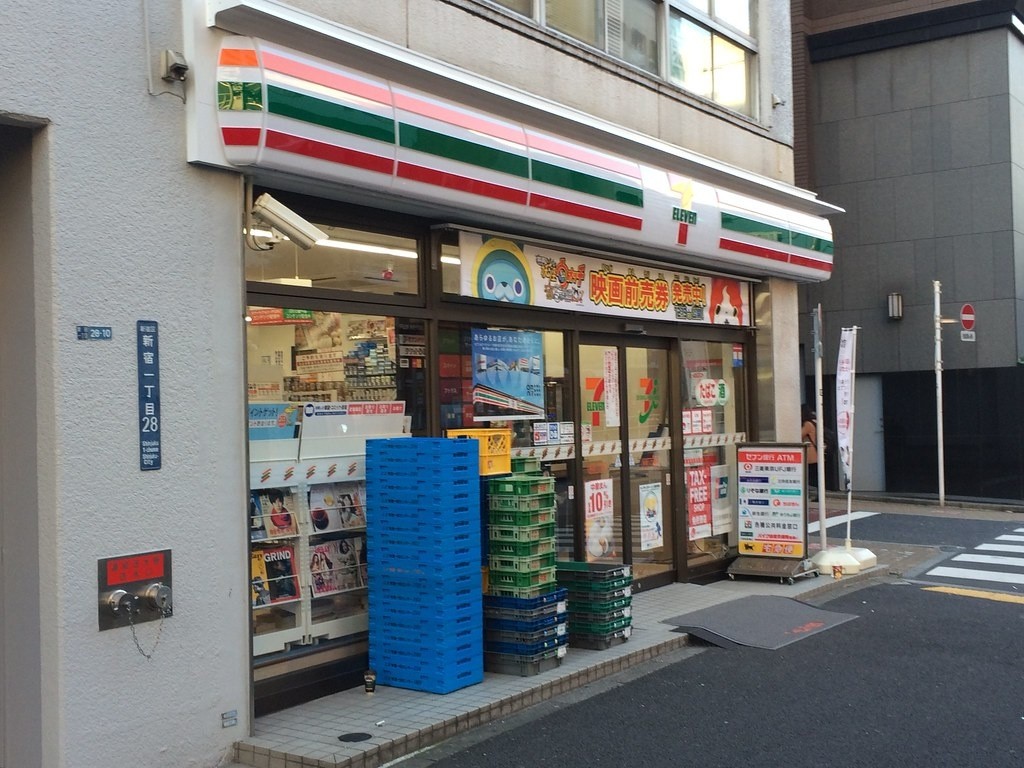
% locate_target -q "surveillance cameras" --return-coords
[251,193,330,252]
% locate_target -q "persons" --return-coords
[268,490,292,530]
[338,495,363,527]
[802,402,828,502]
[310,552,333,591]
[337,541,357,587]
[251,576,270,606]
[274,557,294,599]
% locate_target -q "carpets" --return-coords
[661,593,860,649]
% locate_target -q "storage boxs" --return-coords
[484,472,563,674]
[446,428,513,473]
[365,435,485,693]
[556,562,634,650]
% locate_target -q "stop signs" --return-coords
[960,304,976,331]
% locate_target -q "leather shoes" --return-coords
[814,498,819,504]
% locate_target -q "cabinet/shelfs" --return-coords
[248,433,371,654]
[284,336,396,402]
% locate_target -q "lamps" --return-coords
[887,291,904,320]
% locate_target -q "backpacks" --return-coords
[805,420,834,457]
